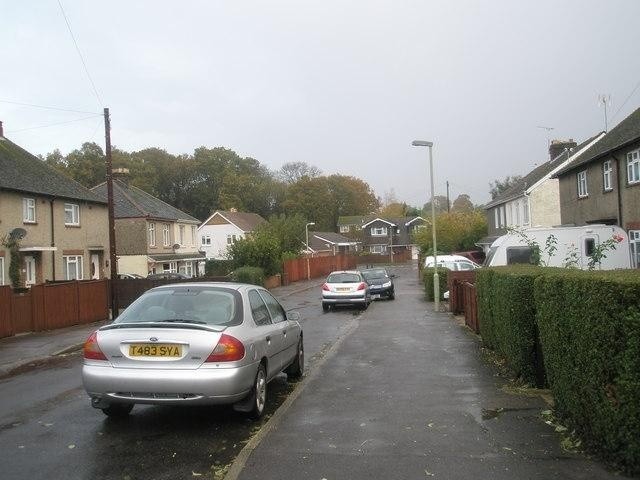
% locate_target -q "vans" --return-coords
[425,251,485,271]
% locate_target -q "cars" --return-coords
[82,282,305,420]
[360,269,396,300]
[322,270,371,310]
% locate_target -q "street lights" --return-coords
[305,222,315,259]
[412,141,438,274]
